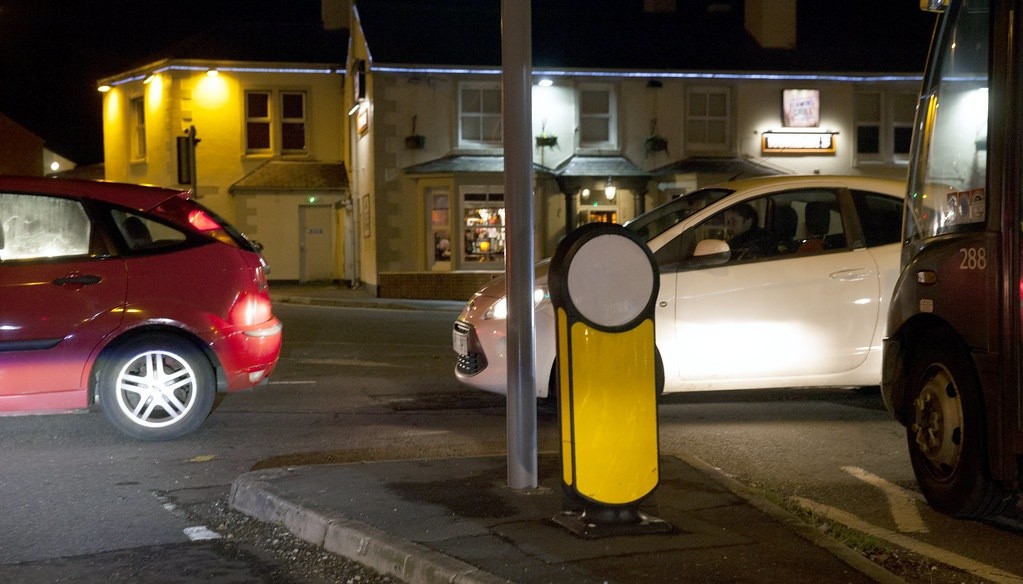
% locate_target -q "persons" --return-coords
[721,204,779,259]
[434,227,500,260]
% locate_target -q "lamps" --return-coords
[605,176,617,201]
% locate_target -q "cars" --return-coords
[0,177,283,440]
[451,172,967,417]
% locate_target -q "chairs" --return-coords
[121,216,154,250]
[801,200,830,251]
[766,205,799,255]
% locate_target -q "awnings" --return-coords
[402,153,556,175]
[554,154,655,176]
[649,156,789,178]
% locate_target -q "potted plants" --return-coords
[536,134,560,152]
[466,212,482,221]
[405,134,426,151]
[645,132,671,158]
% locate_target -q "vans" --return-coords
[880,0,1022,517]
